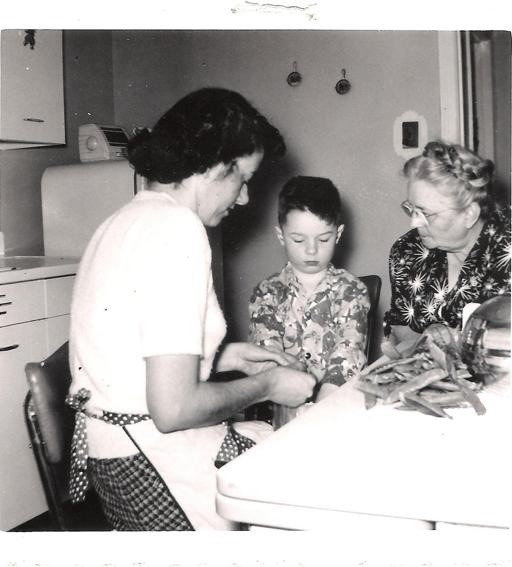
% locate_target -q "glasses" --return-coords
[399,199,465,219]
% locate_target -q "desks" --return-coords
[218,324,508,530]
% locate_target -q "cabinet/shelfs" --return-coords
[0,265,80,532]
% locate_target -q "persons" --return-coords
[65,86,317,528]
[381,141,512,349]
[246,175,371,428]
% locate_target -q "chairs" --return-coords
[23,341,92,526]
[266,268,380,365]
